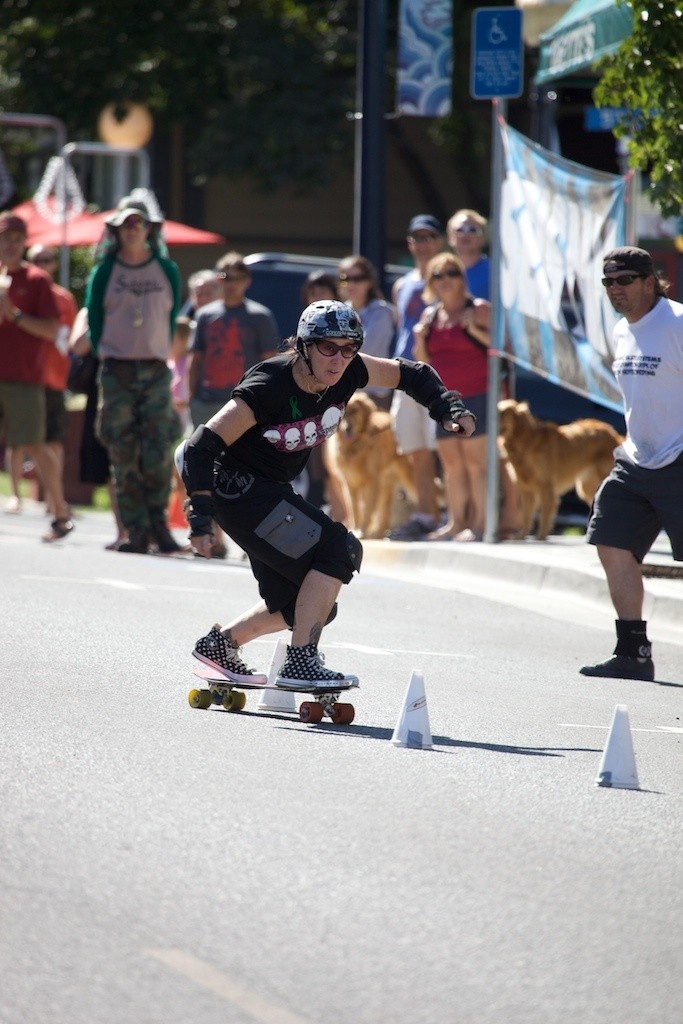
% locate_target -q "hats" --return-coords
[603,246,652,273]
[409,215,440,233]
[104,195,162,236]
[0,213,28,236]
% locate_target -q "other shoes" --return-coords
[428,528,454,540]
[386,520,437,540]
[454,528,484,542]
[44,518,75,539]
[5,497,20,513]
[151,507,178,550]
[119,530,148,553]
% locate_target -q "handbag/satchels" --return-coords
[67,351,99,393]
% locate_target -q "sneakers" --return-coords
[193,624,267,684]
[275,643,359,689]
[579,654,654,681]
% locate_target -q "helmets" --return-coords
[297,300,364,344]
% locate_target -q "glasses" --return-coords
[432,269,461,278]
[409,235,436,241]
[121,220,149,230]
[313,339,360,358]
[602,275,644,286]
[454,226,477,233]
[338,275,365,283]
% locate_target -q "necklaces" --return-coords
[132,267,147,327]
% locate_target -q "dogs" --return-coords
[494,398,626,541]
[322,390,445,540]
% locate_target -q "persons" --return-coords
[186,254,277,557]
[579,246,682,681]
[389,210,520,542]
[0,211,130,549]
[333,257,399,537]
[173,300,477,687]
[301,272,341,524]
[170,272,218,439]
[83,196,184,553]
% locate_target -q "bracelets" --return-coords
[13,311,24,325]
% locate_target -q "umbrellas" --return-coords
[0,195,222,246]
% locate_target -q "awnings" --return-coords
[533,0,634,145]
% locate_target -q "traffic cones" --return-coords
[156,466,191,528]
[593,704,648,791]
[390,674,437,752]
[254,635,302,716]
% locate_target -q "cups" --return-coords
[0,275,12,296]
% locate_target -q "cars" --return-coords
[240,253,420,351]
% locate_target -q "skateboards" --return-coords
[186,669,359,726]
